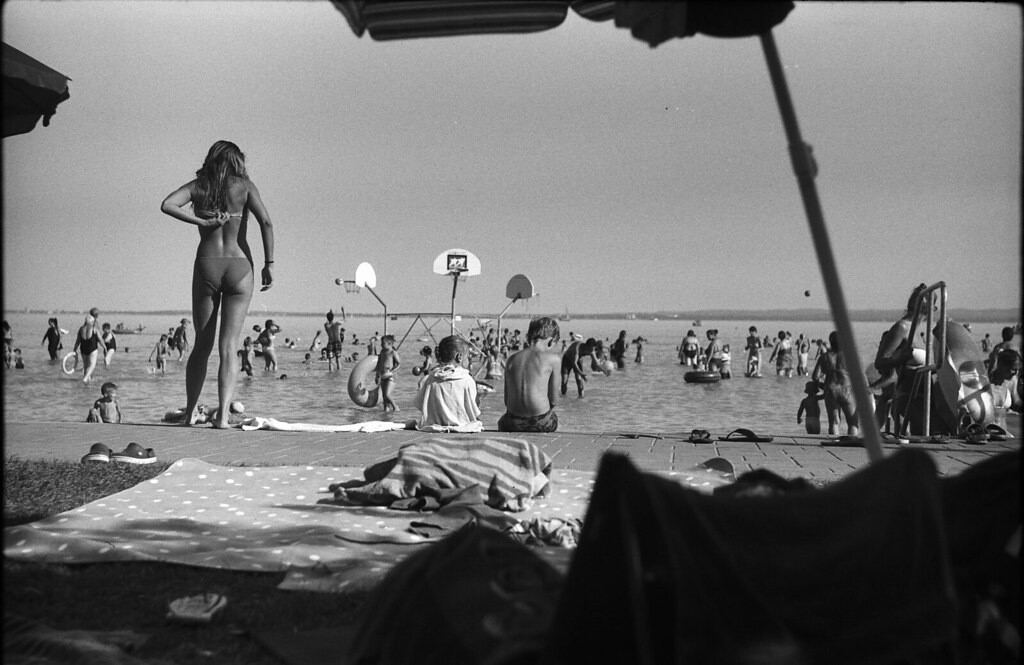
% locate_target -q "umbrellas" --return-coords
[0,38,71,138]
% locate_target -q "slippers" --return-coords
[965,422,988,444]
[820,434,868,448]
[932,430,952,444]
[716,427,774,443]
[985,424,1007,441]
[880,431,910,444]
[688,428,714,444]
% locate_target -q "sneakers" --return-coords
[111,442,158,465]
[81,442,112,465]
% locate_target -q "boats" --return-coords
[111,327,138,335]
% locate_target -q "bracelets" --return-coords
[263,260,275,265]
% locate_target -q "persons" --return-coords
[497,317,561,432]
[414,336,480,433]
[160,140,275,429]
[0,282,1024,437]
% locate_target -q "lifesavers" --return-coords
[62,351,79,375]
[347,355,397,408]
[417,338,428,342]
[684,370,721,383]
